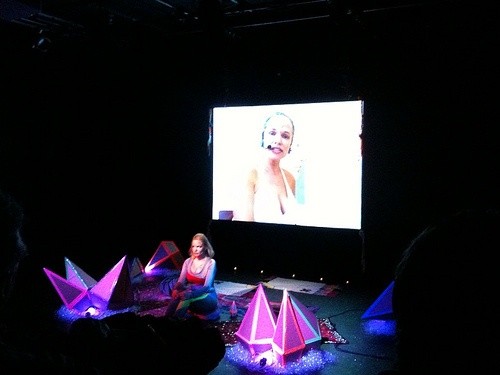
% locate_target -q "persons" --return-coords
[164,234,218,320]
[228,112,299,223]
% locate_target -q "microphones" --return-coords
[263,145,271,149]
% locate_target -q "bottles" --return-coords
[230,301,238,317]
